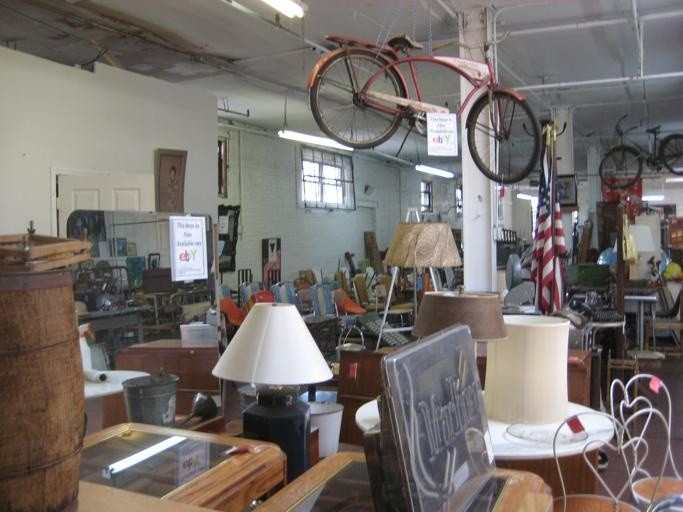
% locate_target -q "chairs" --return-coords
[215,250,462,379]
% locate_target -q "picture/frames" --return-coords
[151,148,188,217]
[557,173,578,207]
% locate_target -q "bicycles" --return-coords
[597,115,683,189]
[306,28,541,184]
[525,119,568,186]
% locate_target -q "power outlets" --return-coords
[380,220,463,328]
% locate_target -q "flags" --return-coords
[530,145,567,317]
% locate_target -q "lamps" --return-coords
[411,138,455,182]
[514,183,533,202]
[259,0,309,22]
[274,94,355,153]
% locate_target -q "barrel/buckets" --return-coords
[1,266,86,512]
[307,400,345,459]
[123,373,182,429]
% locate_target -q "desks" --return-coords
[44,277,683,510]
